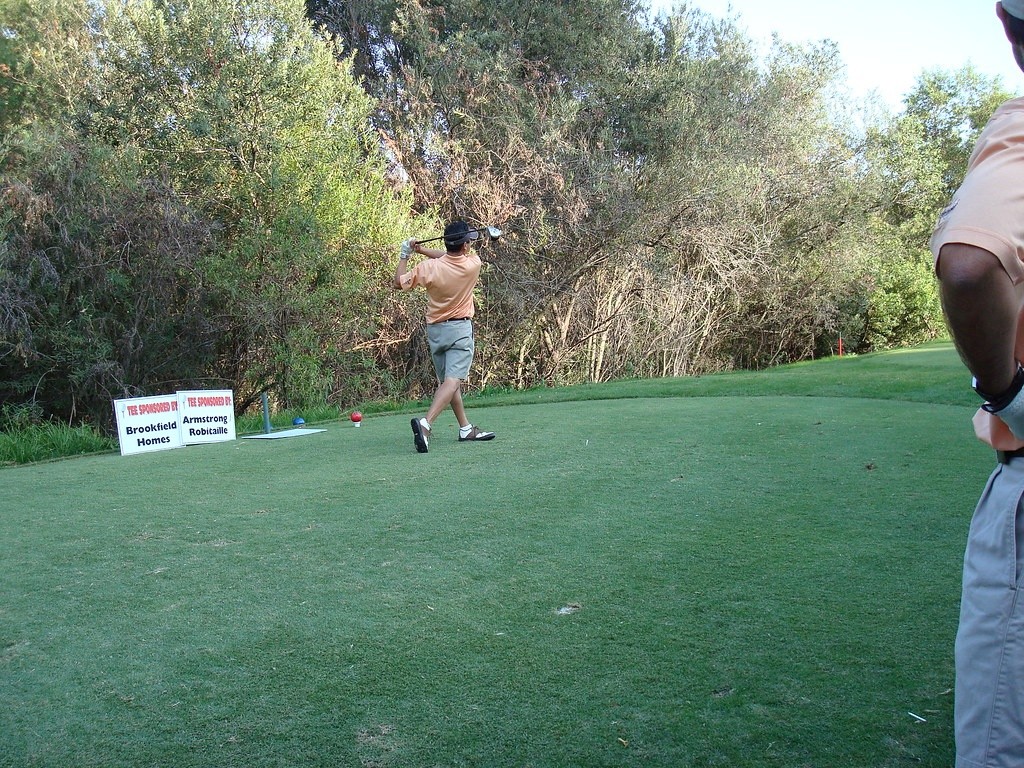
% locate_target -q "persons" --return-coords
[394,220,496,454]
[935,0,1024,768]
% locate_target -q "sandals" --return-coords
[459,424,495,441]
[411,417,434,453]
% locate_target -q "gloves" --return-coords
[981,360,1024,442]
[400,239,414,260]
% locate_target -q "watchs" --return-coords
[399,253,410,260]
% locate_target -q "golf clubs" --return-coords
[416,225,501,245]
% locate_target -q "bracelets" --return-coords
[970,357,1021,403]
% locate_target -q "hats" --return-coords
[445,221,478,246]
[1000,0,1024,20]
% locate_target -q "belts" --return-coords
[437,317,468,323]
[996,446,1024,464]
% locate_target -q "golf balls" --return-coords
[292,417,305,425]
[351,411,362,421]
